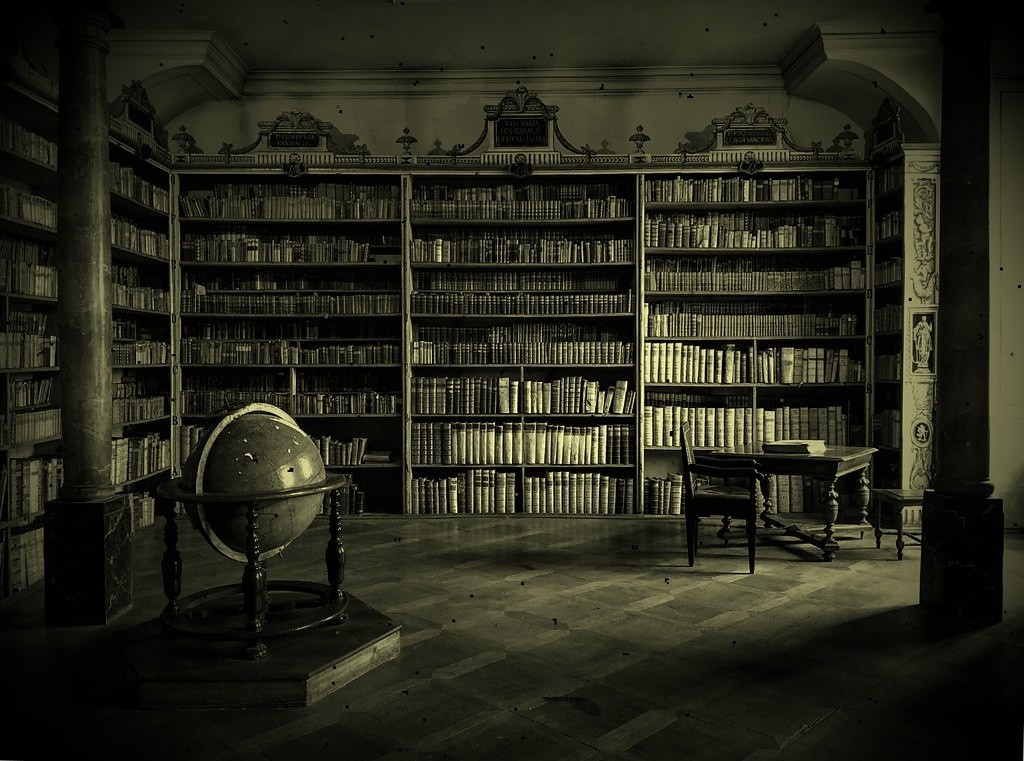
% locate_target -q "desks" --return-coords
[712,440,878,561]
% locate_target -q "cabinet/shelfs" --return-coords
[0,50,941,602]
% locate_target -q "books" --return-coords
[413,322,634,364]
[411,375,637,414]
[411,270,636,314]
[182,225,402,263]
[319,471,366,515]
[179,183,401,219]
[0,114,169,600]
[410,184,632,220]
[181,270,401,314]
[411,227,634,264]
[181,320,399,365]
[308,434,391,465]
[296,373,401,414]
[411,422,636,464]
[181,503,186,515]
[180,424,206,465]
[643,165,903,516]
[411,469,633,514]
[181,371,290,414]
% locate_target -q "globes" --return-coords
[149,402,352,651]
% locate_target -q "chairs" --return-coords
[679,421,766,573]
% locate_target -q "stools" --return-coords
[869,487,924,562]
